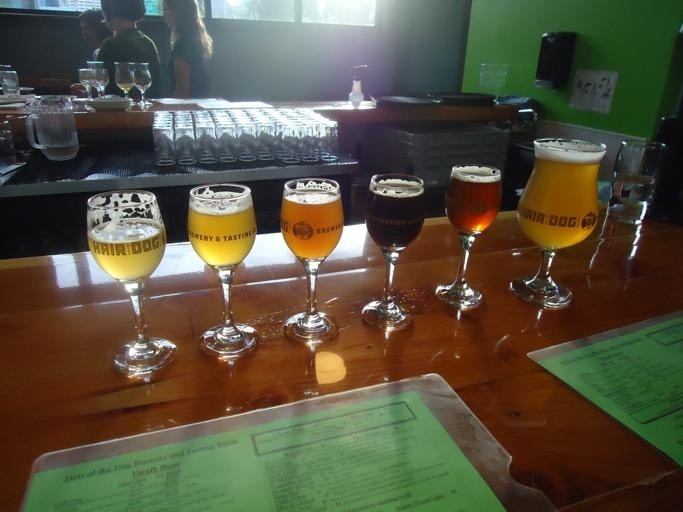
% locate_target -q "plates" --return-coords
[0,86,77,104]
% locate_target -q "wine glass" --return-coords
[83,189,179,377]
[507,139,608,309]
[138,304,569,484]
[183,182,257,357]
[76,60,154,112]
[279,176,345,344]
[434,163,504,310]
[360,171,425,331]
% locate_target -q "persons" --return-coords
[158,0,214,99]
[77,9,113,60]
[70,0,166,100]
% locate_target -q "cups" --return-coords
[25,95,79,161]
[0,64,20,98]
[0,120,17,164]
[580,218,642,295]
[606,136,667,228]
[150,106,341,166]
